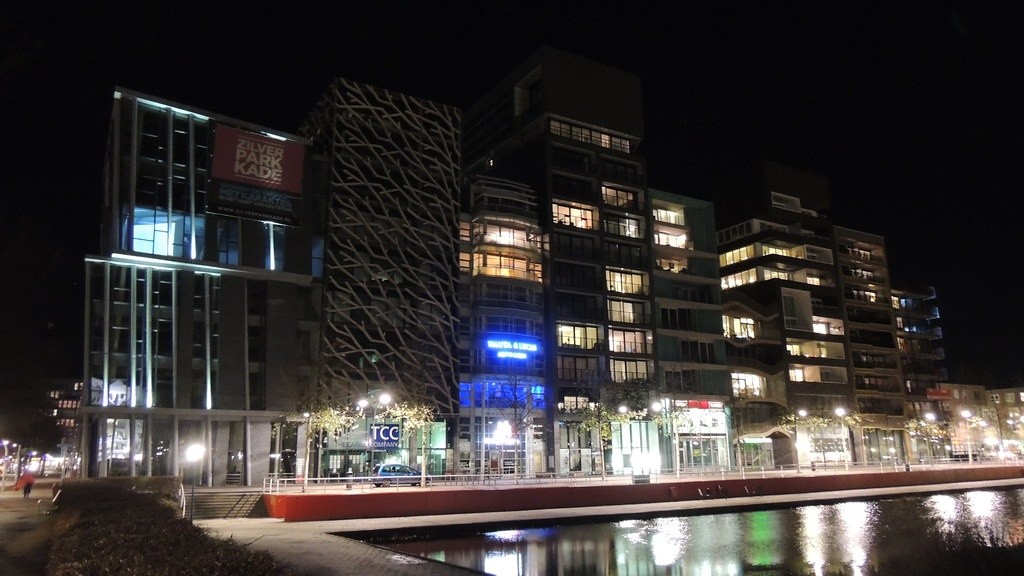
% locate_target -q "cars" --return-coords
[370,463,433,488]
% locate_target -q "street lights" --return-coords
[794,410,807,472]
[357,392,391,484]
[924,413,937,466]
[184,443,206,527]
[651,401,675,472]
[834,406,848,471]
[960,410,975,465]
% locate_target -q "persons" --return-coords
[20,469,35,499]
